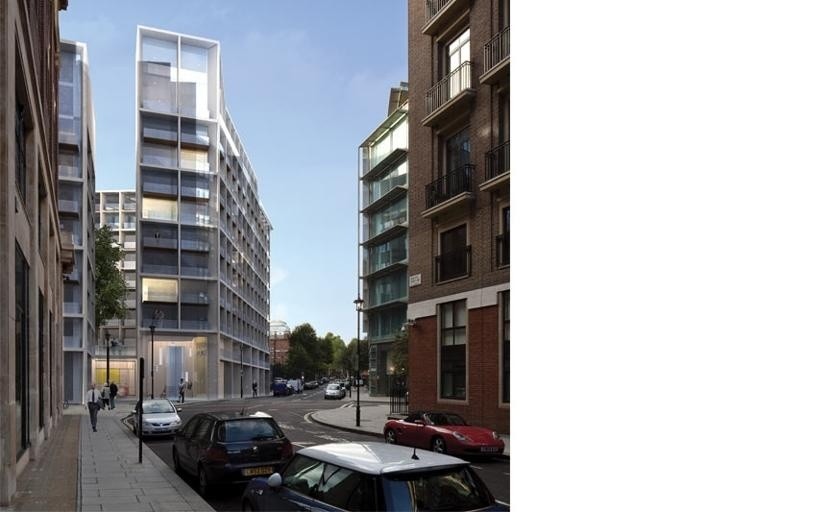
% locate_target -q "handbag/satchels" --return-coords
[101,388,105,398]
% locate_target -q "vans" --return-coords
[172,407,294,502]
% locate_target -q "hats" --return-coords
[104,383,108,386]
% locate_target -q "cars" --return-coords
[383,409,507,460]
[272,375,364,399]
[237,440,508,512]
[131,398,184,438]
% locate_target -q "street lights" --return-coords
[149,322,157,400]
[105,329,112,386]
[353,293,365,428]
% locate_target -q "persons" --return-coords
[110,379,118,409]
[270,379,278,397]
[155,309,165,328]
[85,383,103,432]
[176,377,187,403]
[154,231,161,243]
[100,383,111,410]
[252,380,257,397]
[278,381,286,397]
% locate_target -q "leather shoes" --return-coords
[92,406,116,433]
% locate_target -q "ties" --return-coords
[92,391,94,403]
